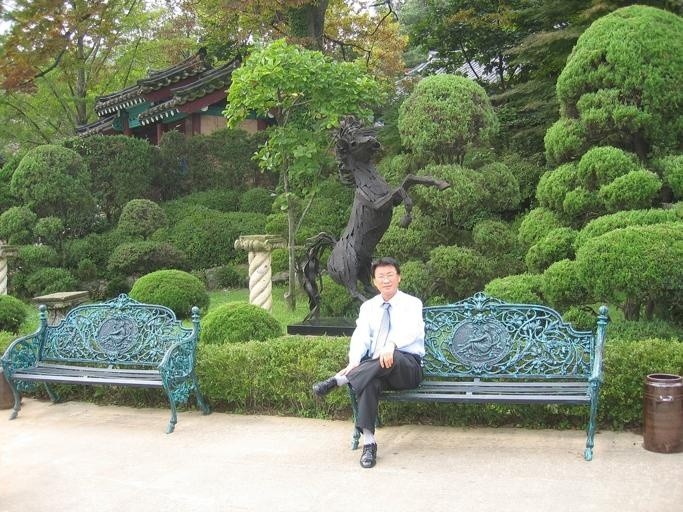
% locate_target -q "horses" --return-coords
[300,116,452,323]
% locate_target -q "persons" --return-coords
[313,256,425,467]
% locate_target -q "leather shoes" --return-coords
[311,376,337,397]
[359,443,377,468]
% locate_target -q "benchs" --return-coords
[346,291,608,461]
[0,294,212,435]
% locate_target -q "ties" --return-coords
[370,303,390,360]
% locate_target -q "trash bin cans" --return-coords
[643,373,682,454]
